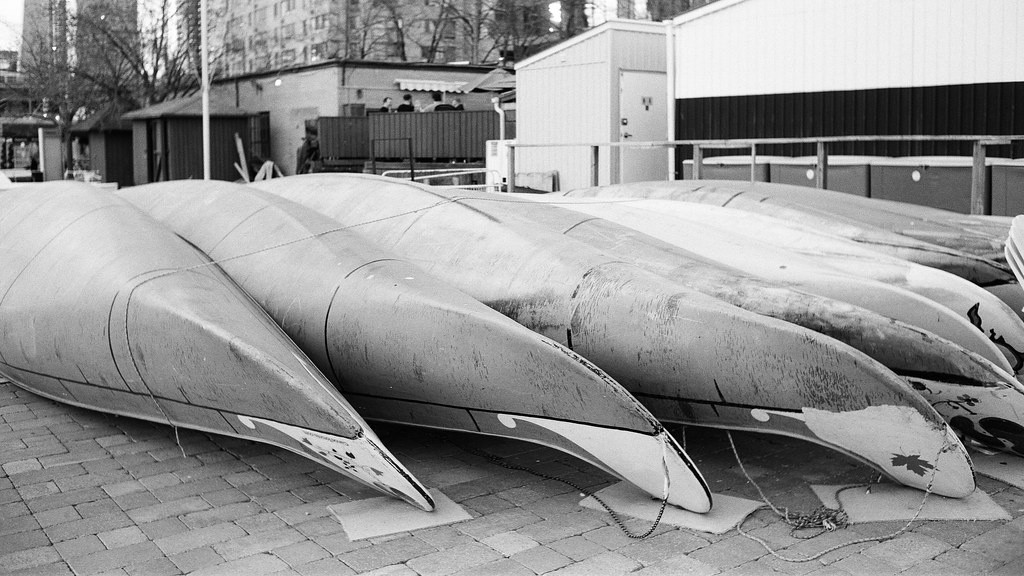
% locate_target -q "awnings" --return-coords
[393,79,469,94]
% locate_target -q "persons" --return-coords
[420,92,442,112]
[296,126,319,174]
[81,142,91,170]
[452,97,464,110]
[395,94,415,112]
[380,97,392,113]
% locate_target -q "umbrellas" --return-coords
[458,68,516,94]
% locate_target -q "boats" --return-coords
[2,182,437,512]
[117,172,1024,514]
